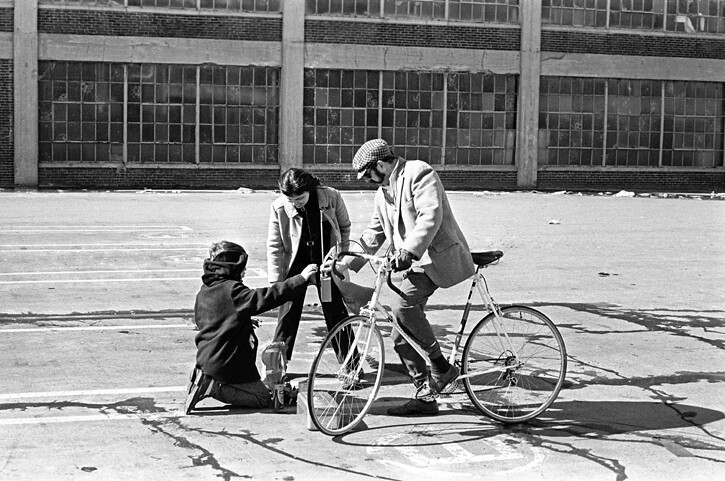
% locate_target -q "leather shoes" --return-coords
[415,364,459,399]
[387,399,439,417]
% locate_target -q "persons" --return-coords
[266,168,363,387]
[184,241,318,413]
[320,138,477,417]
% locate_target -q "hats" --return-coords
[352,139,391,179]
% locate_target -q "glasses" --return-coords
[365,170,372,178]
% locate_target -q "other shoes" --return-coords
[184,368,213,415]
[338,366,364,389]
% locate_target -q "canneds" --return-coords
[274,384,284,411]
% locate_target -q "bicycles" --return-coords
[306,239,568,436]
